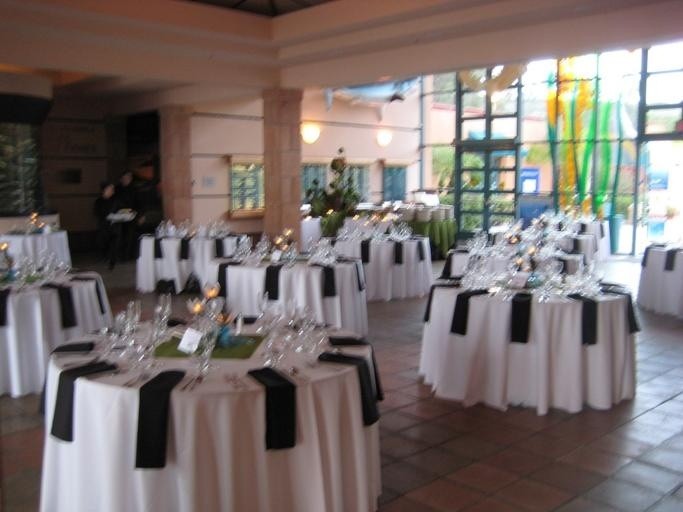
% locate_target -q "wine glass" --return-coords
[157,218,232,241]
[101,300,156,376]
[280,236,336,264]
[233,234,273,267]
[338,215,413,244]
[155,293,174,335]
[257,301,330,373]
[188,317,219,377]
[461,210,604,298]
[15,251,70,287]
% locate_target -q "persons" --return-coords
[94,160,162,274]
